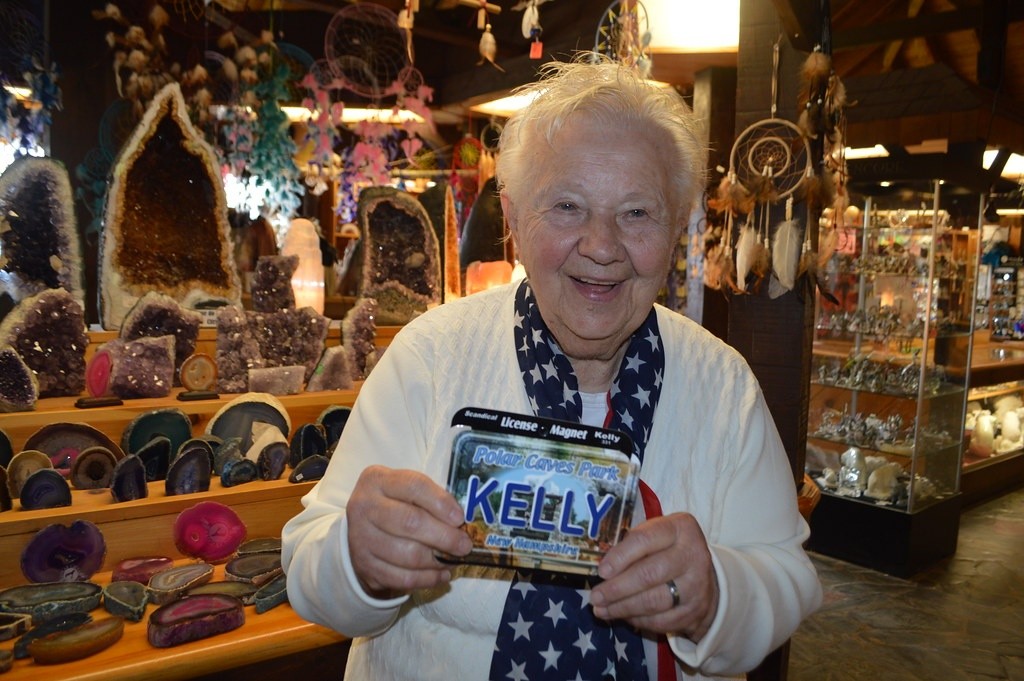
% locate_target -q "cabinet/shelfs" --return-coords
[799,183,1024,577]
[1,328,366,681]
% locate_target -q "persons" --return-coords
[277,42,824,681]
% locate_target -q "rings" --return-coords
[666,579,681,608]
